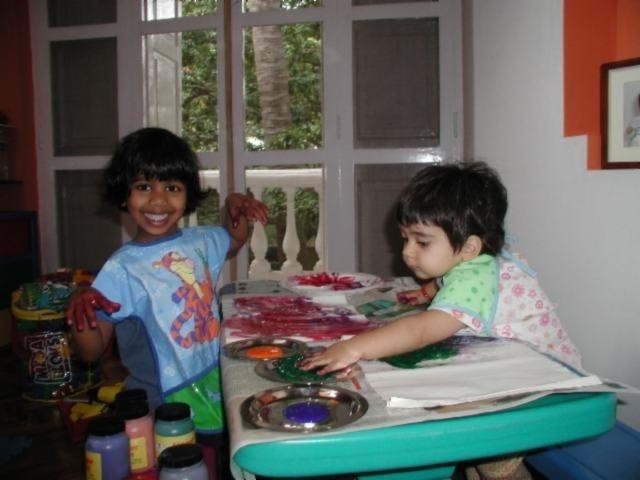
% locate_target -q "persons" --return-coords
[296,160,584,480]
[62,125,271,453]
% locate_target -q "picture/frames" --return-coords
[599,57,640,170]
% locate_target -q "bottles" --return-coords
[82,386,210,480]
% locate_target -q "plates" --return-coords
[254,356,361,385]
[277,270,385,297]
[240,382,369,434]
[222,336,307,363]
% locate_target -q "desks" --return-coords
[218,276,620,479]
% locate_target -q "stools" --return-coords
[522,420,639,480]
